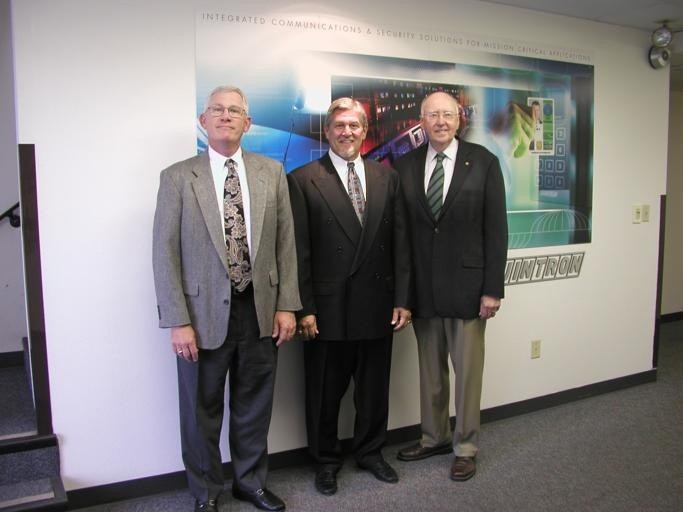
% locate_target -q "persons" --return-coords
[393,91,508,480]
[153,86,302,512]
[286,98,413,496]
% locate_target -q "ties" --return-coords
[223,159,252,293]
[426,154,449,221]
[347,162,365,228]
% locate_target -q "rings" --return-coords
[296,330,302,335]
[408,320,411,324]
[179,351,183,354]
[491,311,495,317]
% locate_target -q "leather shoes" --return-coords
[360,458,398,482]
[315,469,337,495]
[233,485,286,512]
[451,455,476,481]
[195,496,218,512]
[398,442,453,460]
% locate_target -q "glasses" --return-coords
[208,105,245,117]
